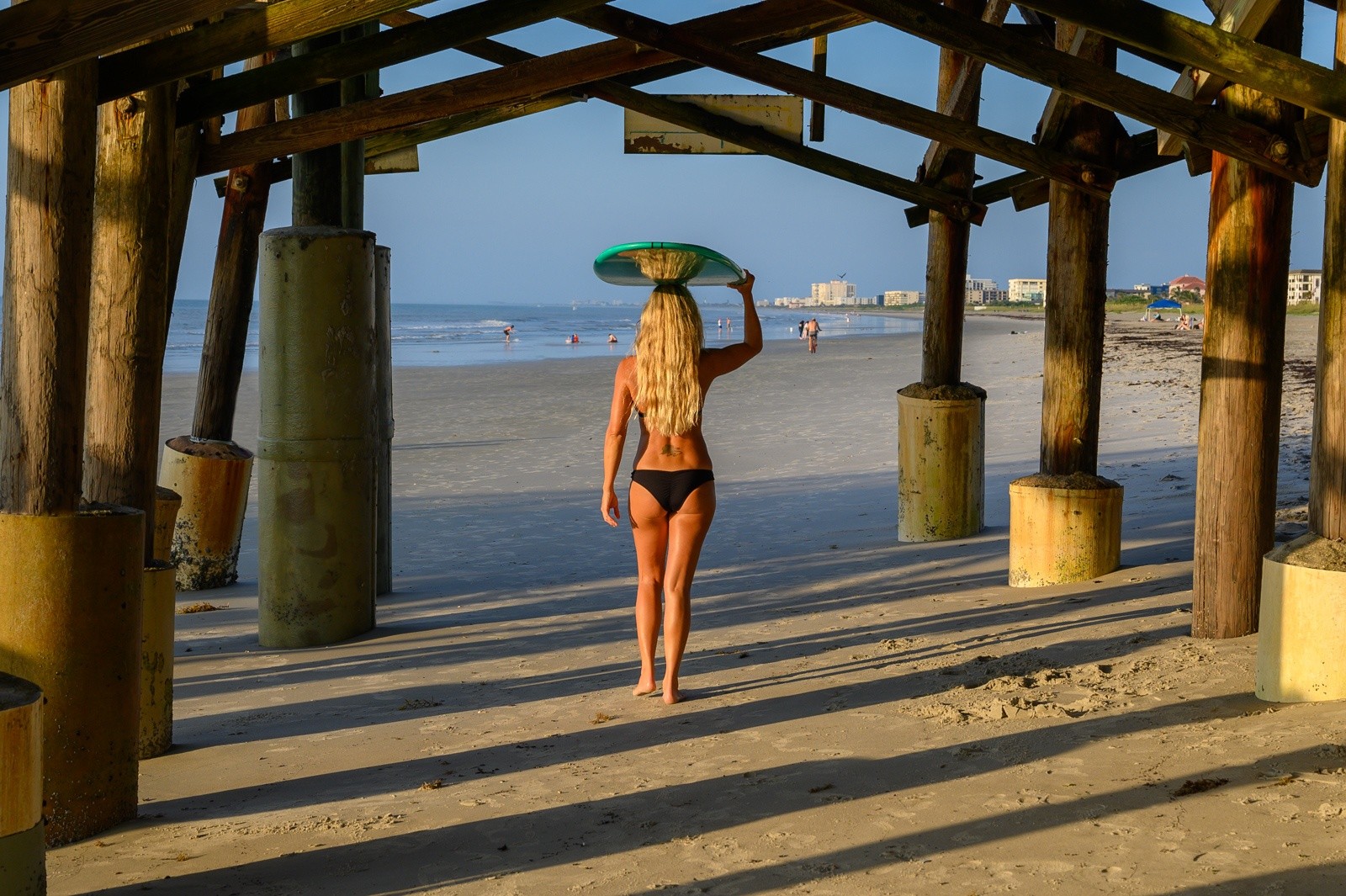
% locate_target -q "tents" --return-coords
[1146,298,1182,322]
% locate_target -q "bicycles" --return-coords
[810,329,820,354]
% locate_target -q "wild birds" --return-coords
[836,272,847,279]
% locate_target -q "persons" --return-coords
[600,282,764,705]
[504,325,514,342]
[607,334,617,342]
[570,334,579,343]
[1193,317,1204,330]
[798,320,805,340]
[1154,311,1161,320]
[803,321,809,339]
[1176,315,1192,331]
[807,318,822,353]
[718,318,722,329]
[726,317,731,330]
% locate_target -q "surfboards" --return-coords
[592,241,748,287]
